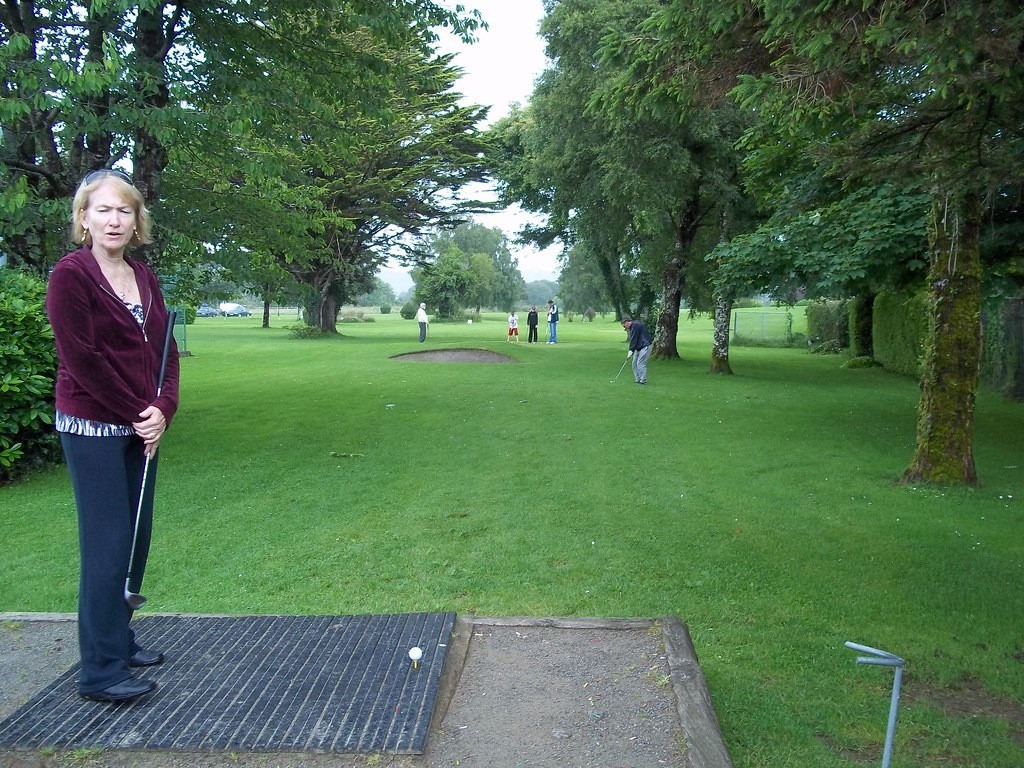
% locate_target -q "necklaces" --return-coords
[99,263,134,299]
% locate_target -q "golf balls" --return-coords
[409,646,423,660]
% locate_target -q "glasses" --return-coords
[84,169,134,187]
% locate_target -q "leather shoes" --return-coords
[128,646,164,665]
[78,676,157,702]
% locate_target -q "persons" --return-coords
[526,306,538,343]
[418,303,429,343]
[620,318,652,385]
[546,299,558,344]
[46,169,181,703]
[508,311,520,343]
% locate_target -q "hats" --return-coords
[621,313,630,323]
[420,303,427,309]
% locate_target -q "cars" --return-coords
[195,307,217,318]
[220,303,252,317]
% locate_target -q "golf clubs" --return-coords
[123,310,178,612]
[609,359,628,383]
[545,313,550,344]
[526,324,530,344]
